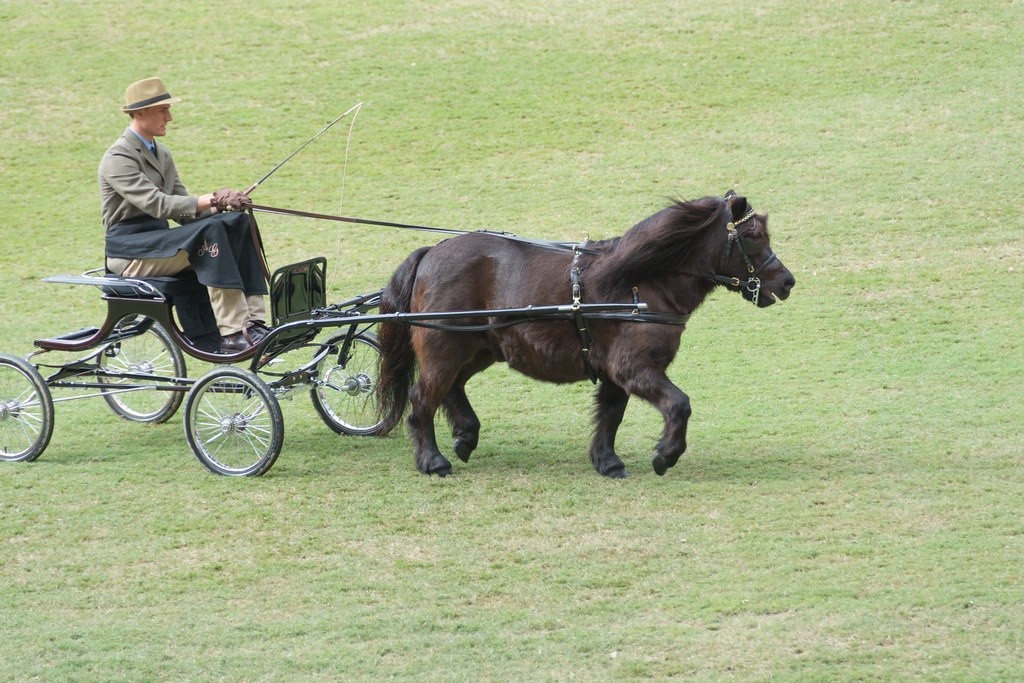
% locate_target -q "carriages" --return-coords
[0,189,797,480]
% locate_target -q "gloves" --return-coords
[209,188,252,213]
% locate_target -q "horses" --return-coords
[375,190,796,477]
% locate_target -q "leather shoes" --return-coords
[220,328,265,353]
[253,324,270,335]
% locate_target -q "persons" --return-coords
[97,77,274,354]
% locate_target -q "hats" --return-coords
[121,77,183,113]
